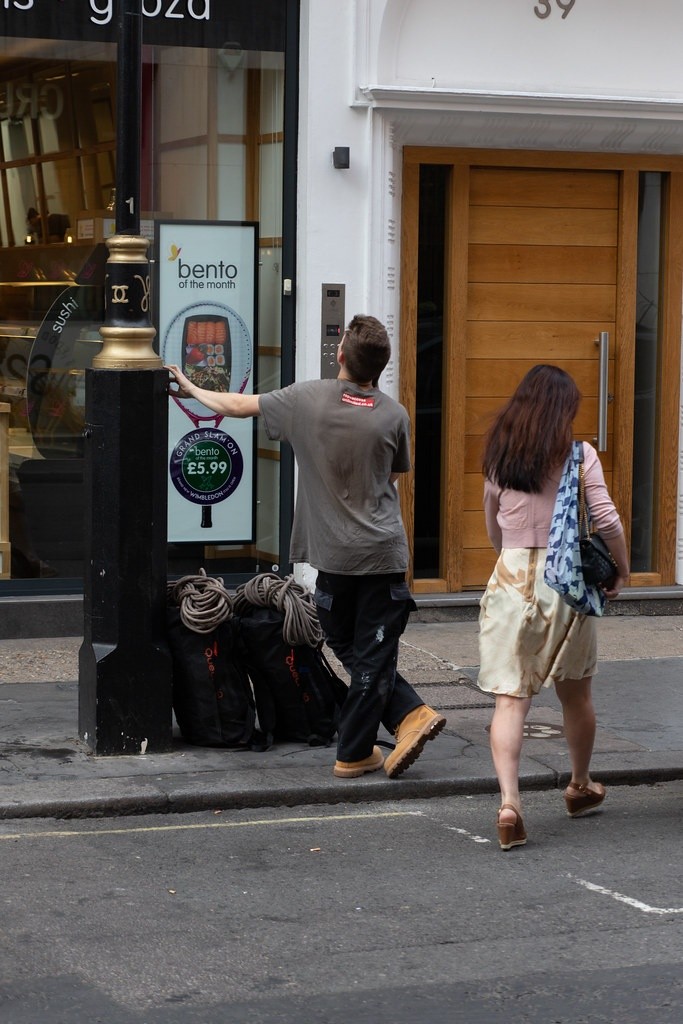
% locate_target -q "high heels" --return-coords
[565,782,606,817]
[496,804,527,849]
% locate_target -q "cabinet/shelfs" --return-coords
[2,45,276,458]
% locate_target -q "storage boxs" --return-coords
[75,209,173,247]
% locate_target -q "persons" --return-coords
[476,364,631,848]
[163,314,448,777]
[27,208,70,243]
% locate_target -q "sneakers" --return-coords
[334,745,385,777]
[383,704,446,777]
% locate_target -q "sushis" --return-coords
[205,344,226,367]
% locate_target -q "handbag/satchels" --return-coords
[544,441,606,620]
[95,599,349,758]
[575,441,620,588]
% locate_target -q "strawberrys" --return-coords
[187,343,207,365]
